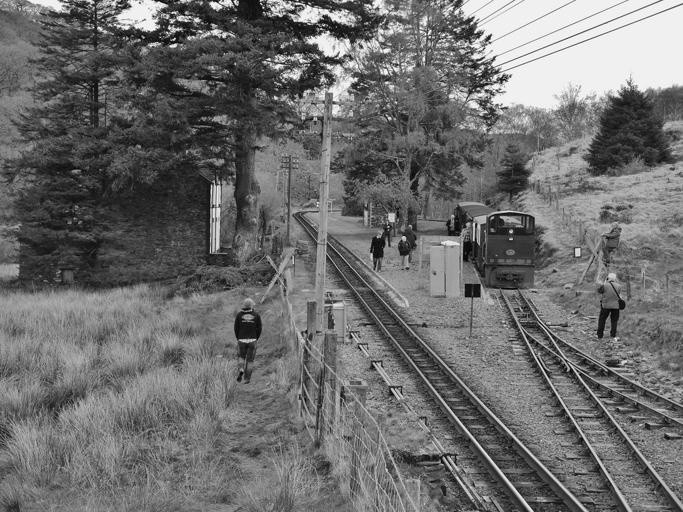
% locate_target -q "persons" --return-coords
[445,214,458,236]
[402,223,417,264]
[233,298,263,385]
[490,216,504,233]
[597,272,623,339]
[397,235,412,270]
[459,222,473,262]
[381,221,393,248]
[368,230,387,272]
[600,222,622,266]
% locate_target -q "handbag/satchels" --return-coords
[619,300,625,309]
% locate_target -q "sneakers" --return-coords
[237,372,250,384]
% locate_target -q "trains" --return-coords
[456,201,536,288]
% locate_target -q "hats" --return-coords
[244,298,256,307]
